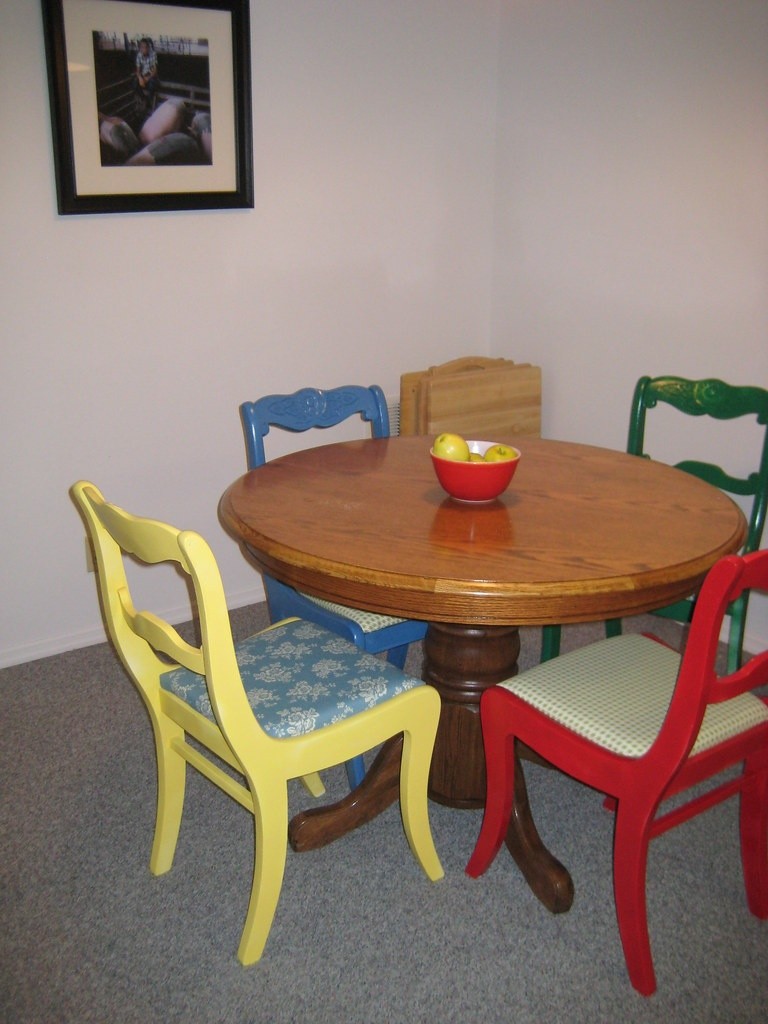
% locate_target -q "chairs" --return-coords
[239,383,428,788]
[540,376,768,674]
[74,481,445,966]
[465,549,768,994]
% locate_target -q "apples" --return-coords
[433,432,518,462]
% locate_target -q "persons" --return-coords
[131,38,159,104]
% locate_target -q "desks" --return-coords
[216,434,749,915]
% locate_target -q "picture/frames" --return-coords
[40,0,254,216]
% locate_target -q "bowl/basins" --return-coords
[430,441,520,502]
